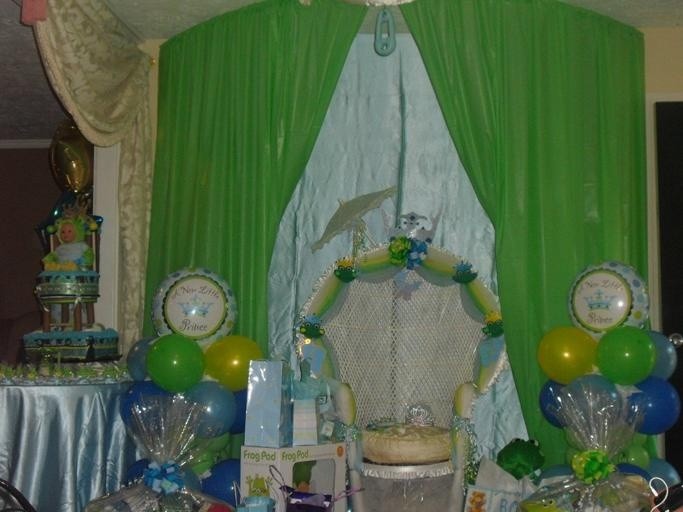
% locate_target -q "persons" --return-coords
[40,213,95,275]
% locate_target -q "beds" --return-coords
[127,2,658,512]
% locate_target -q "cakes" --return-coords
[361,422,448,463]
[22,214,119,363]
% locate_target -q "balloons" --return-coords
[118,331,264,452]
[567,261,650,341]
[537,326,682,493]
[150,268,239,344]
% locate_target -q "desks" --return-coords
[1,363,152,511]
